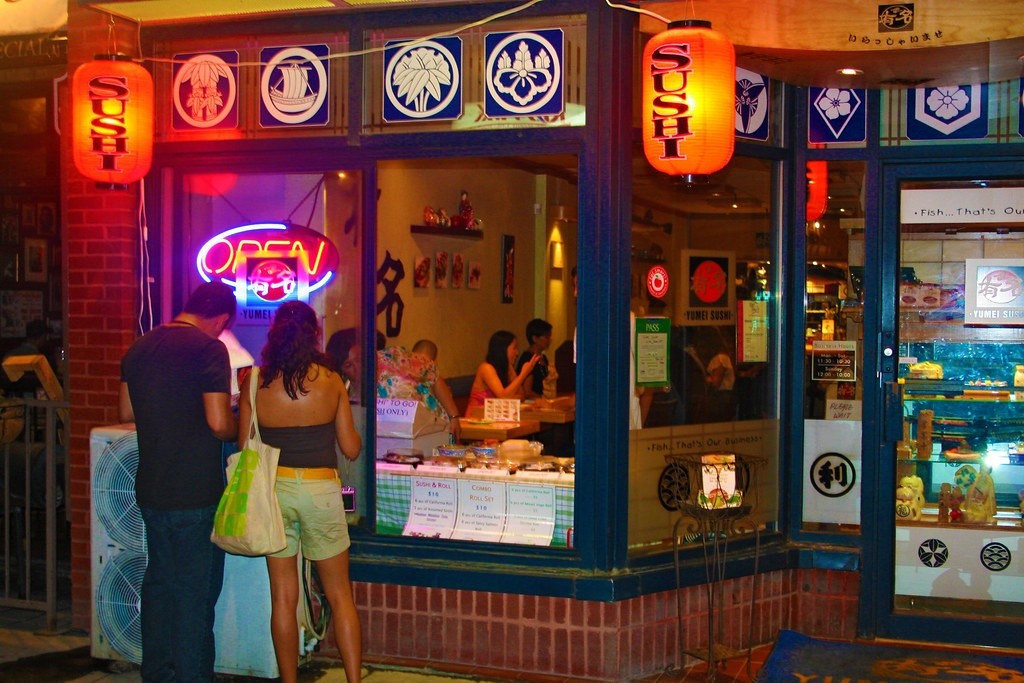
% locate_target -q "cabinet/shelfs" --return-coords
[897,396,1024,466]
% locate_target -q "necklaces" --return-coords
[170,320,195,326]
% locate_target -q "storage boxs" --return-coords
[377,430,450,464]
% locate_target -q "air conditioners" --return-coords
[88,425,313,678]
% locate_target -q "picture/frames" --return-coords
[23,238,49,284]
[499,234,516,303]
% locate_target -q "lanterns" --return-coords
[74,55,153,191]
[642,18,736,191]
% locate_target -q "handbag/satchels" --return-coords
[211,365,287,557]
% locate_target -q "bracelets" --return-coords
[450,415,460,419]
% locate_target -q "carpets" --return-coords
[754,628,1024,683]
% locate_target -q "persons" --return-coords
[238,300,363,683]
[118,281,238,683]
[3,320,50,399]
[328,316,736,446]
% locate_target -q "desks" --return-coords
[457,419,541,440]
[519,403,574,422]
